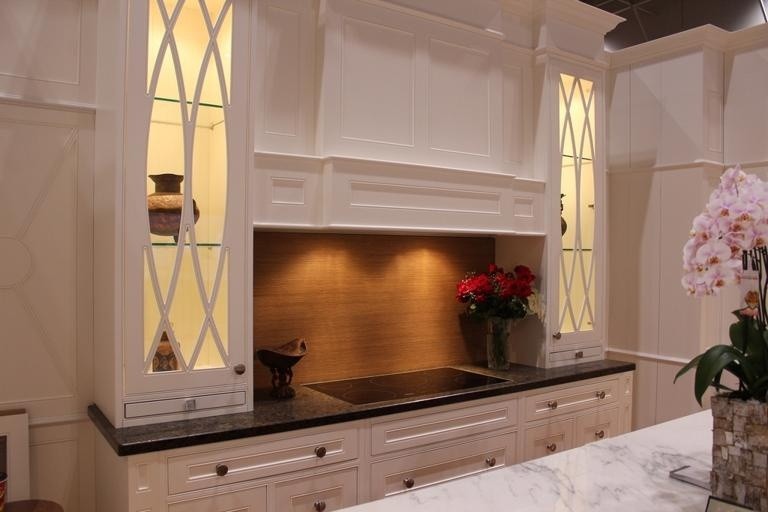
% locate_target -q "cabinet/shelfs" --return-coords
[519,371,634,464]
[89,0,255,429]
[496,54,607,368]
[364,391,520,503]
[91,417,365,512]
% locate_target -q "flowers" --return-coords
[672,162,768,399]
[456,264,536,365]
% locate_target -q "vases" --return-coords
[710,392,768,511]
[144,173,202,235]
[559,193,567,236]
[485,318,512,372]
[152,330,179,372]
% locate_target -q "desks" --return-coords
[350,408,716,512]
